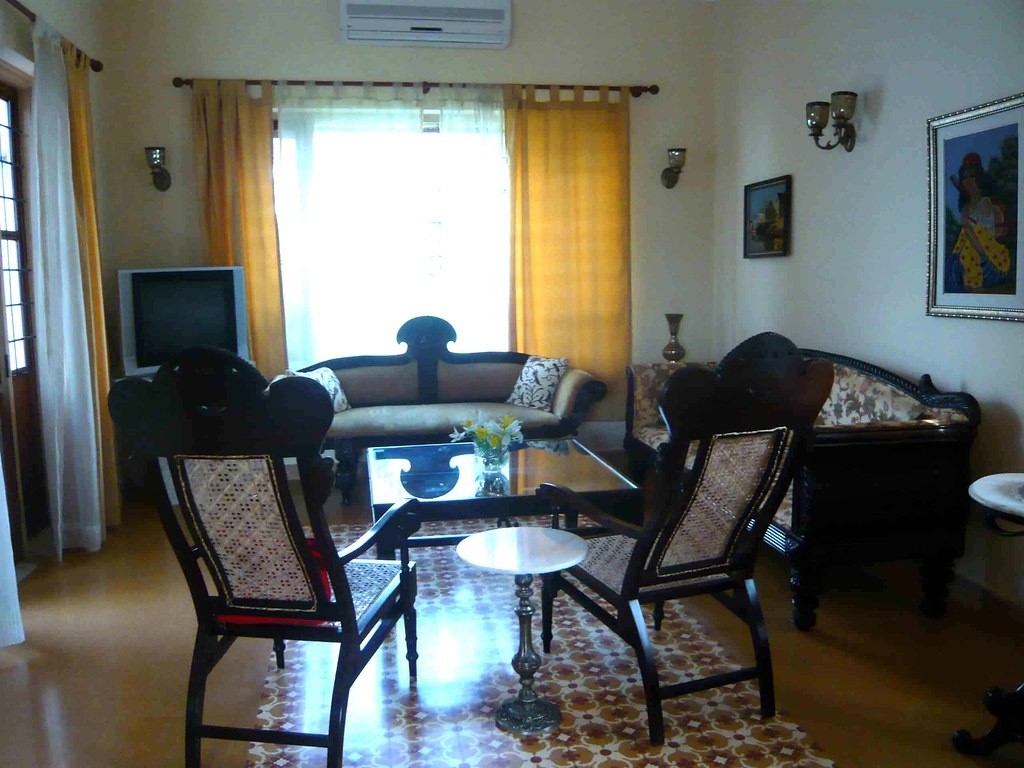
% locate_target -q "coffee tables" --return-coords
[366,438,640,560]
[456,526,589,736]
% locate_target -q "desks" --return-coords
[953,472,1024,768]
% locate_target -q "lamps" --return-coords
[661,148,687,189]
[144,146,171,191]
[806,91,858,153]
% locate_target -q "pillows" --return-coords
[289,366,348,413]
[505,355,569,412]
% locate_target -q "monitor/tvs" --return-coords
[118,266,248,378]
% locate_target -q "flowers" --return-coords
[449,408,530,466]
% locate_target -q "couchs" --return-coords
[270,316,607,506]
[623,348,983,634]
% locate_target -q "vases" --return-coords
[662,313,686,363]
[474,458,511,498]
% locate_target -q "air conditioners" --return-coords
[339,0,512,50]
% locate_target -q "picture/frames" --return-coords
[743,175,792,259]
[927,91,1024,321]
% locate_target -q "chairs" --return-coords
[108,345,422,768]
[539,412,818,745]
